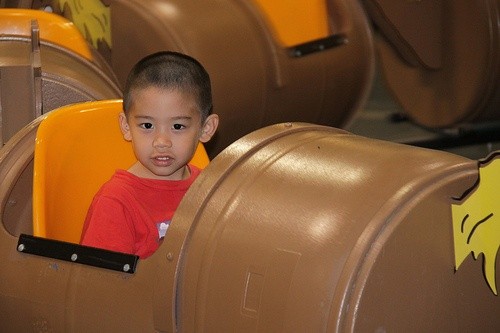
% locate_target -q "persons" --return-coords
[78,50,219,261]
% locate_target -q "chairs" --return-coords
[0,8,95,64]
[32,98,211,245]
